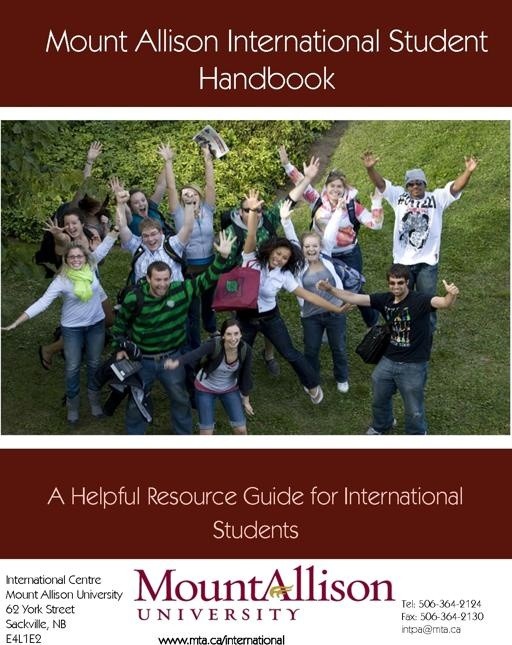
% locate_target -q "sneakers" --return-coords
[262,349,280,377]
[337,381,349,394]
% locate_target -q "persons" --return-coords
[1,126,479,438]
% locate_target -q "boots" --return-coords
[67,394,79,422]
[89,390,103,416]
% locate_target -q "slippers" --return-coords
[333,258,366,294]
[309,385,324,405]
[39,345,52,370]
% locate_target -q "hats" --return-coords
[404,168,427,188]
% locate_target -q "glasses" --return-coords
[242,207,261,214]
[407,180,422,187]
[388,281,405,285]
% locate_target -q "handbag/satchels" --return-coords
[211,266,260,312]
[355,326,391,365]
